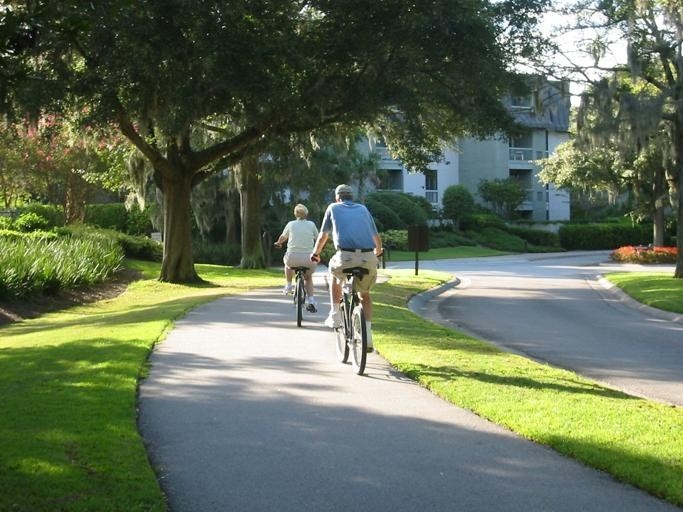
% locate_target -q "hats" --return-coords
[335,184,353,195]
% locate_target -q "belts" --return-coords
[341,248,373,252]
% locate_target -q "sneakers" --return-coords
[366,339,372,352]
[283,288,292,296]
[325,311,342,328]
[309,301,318,313]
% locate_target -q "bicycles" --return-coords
[310,248,384,376]
[275,242,318,327]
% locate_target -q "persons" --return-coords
[310,184,383,353]
[274,203,319,313]
